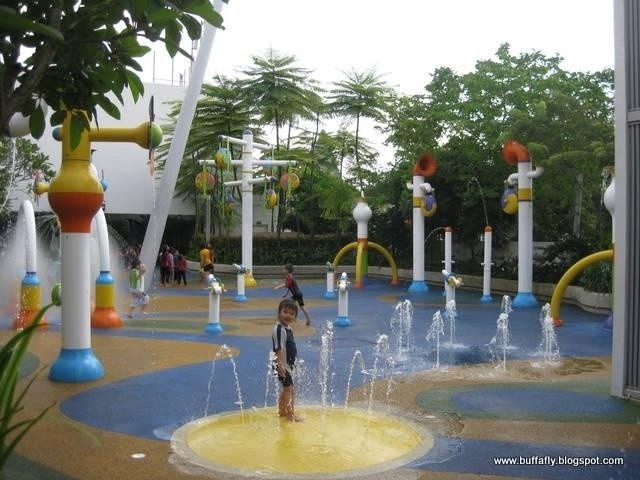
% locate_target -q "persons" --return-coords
[271,299,305,423]
[158,244,188,286]
[274,264,311,326]
[199,242,214,274]
[132,242,141,256]
